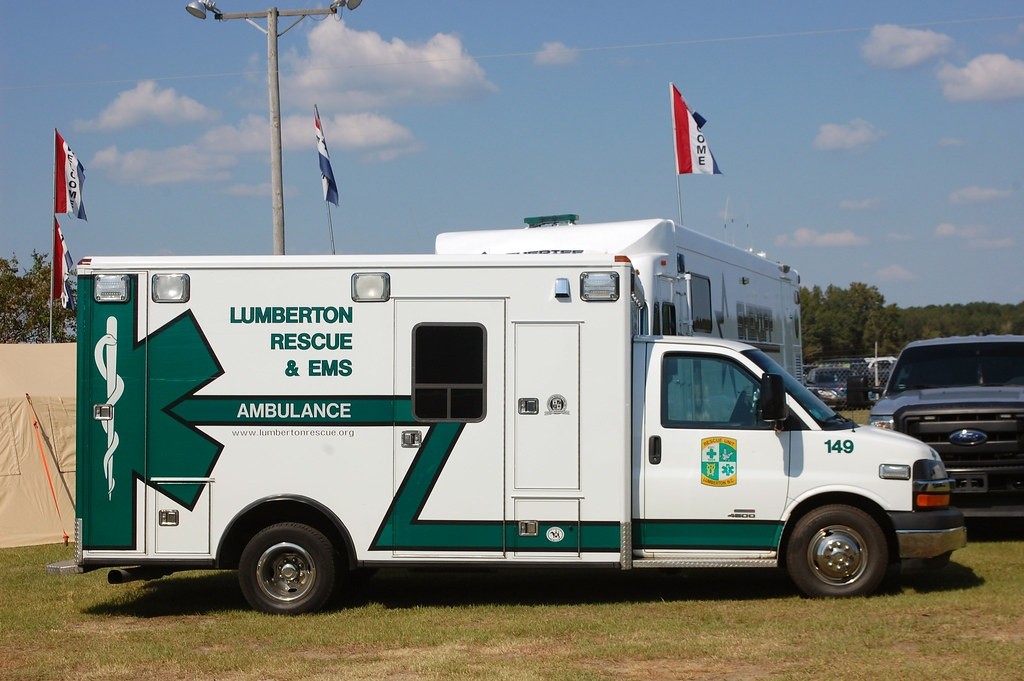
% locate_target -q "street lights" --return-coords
[185,0,363,255]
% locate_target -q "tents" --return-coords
[0,343,76,548]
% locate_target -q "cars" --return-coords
[803,355,897,409]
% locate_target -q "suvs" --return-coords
[868,332,1024,526]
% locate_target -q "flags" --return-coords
[53,216,75,311]
[670,82,722,174]
[55,128,88,222]
[314,103,338,206]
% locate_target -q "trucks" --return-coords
[434,213,804,387]
[71,253,969,616]
